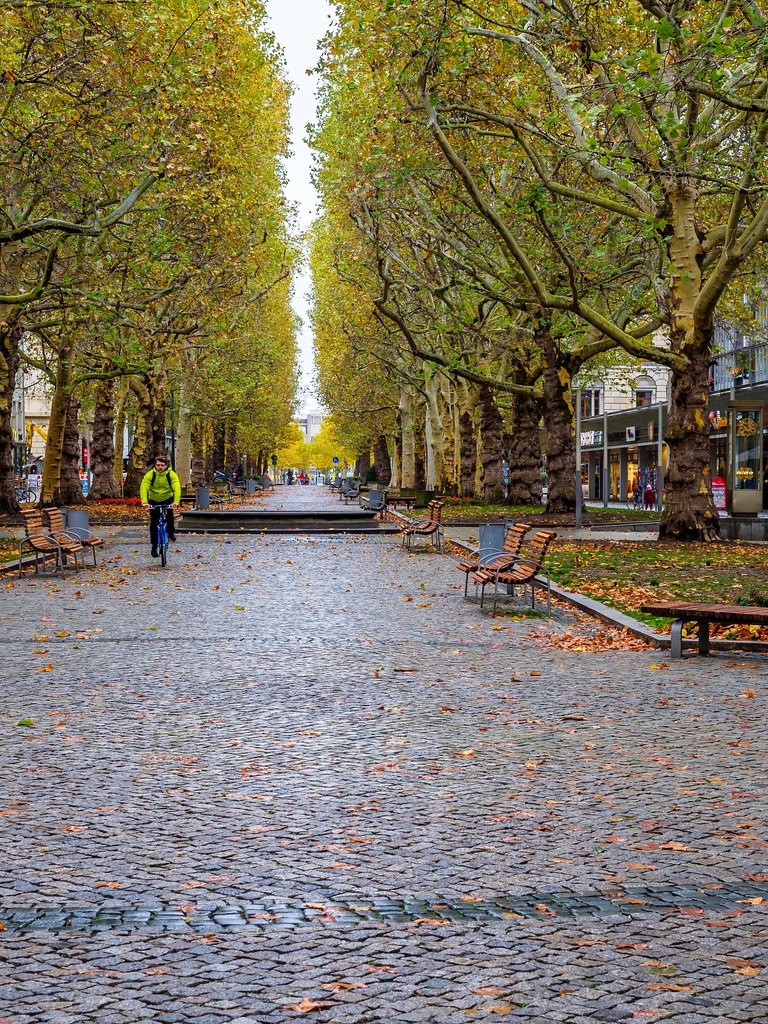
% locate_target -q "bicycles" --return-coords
[145,505,177,567]
[627,483,643,511]
[16,472,36,503]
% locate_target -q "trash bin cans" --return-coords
[477,519,513,565]
[335,477,342,485]
[62,508,89,540]
[263,478,270,489]
[196,488,209,509]
[247,480,255,494]
[369,489,380,508]
[345,477,351,487]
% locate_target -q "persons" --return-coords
[140,456,181,558]
[645,485,655,509]
[264,468,309,484]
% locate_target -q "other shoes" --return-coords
[169,534,176,540]
[151,552,157,557]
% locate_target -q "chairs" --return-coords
[470,529,556,618]
[397,500,438,547]
[331,476,362,504]
[42,506,104,571]
[19,509,83,579]
[456,523,531,604]
[407,502,445,555]
[367,489,389,519]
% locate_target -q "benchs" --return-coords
[641,601,768,658]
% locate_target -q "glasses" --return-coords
[155,464,166,466]
